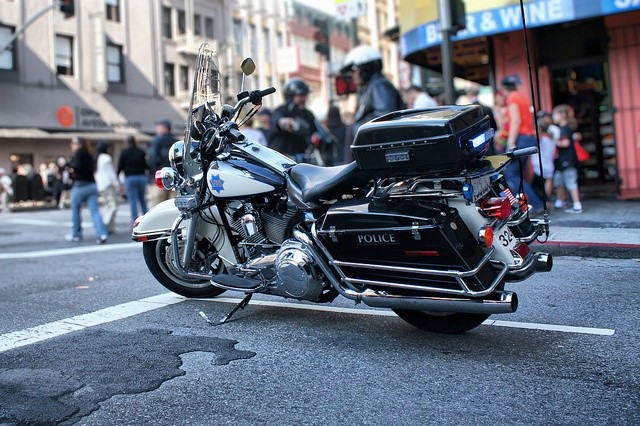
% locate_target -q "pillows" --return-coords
[283,80,308,98]
[338,45,381,78]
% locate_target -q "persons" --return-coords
[255,109,280,143]
[323,107,353,148]
[0,168,15,214]
[268,78,318,165]
[529,117,554,213]
[463,82,497,153]
[68,134,109,244]
[490,87,509,152]
[405,86,439,108]
[338,44,405,160]
[52,155,72,208]
[237,112,268,147]
[500,78,544,213]
[537,110,560,139]
[94,139,122,236]
[12,162,38,182]
[40,162,57,191]
[551,104,584,215]
[146,118,179,207]
[116,134,149,224]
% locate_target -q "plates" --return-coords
[575,93,616,185]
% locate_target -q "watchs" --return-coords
[133,1,552,334]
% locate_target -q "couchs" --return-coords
[337,76,357,95]
[58,0,74,19]
[314,18,328,60]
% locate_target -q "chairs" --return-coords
[555,199,567,208]
[95,233,106,244]
[573,200,583,211]
[64,234,80,242]
